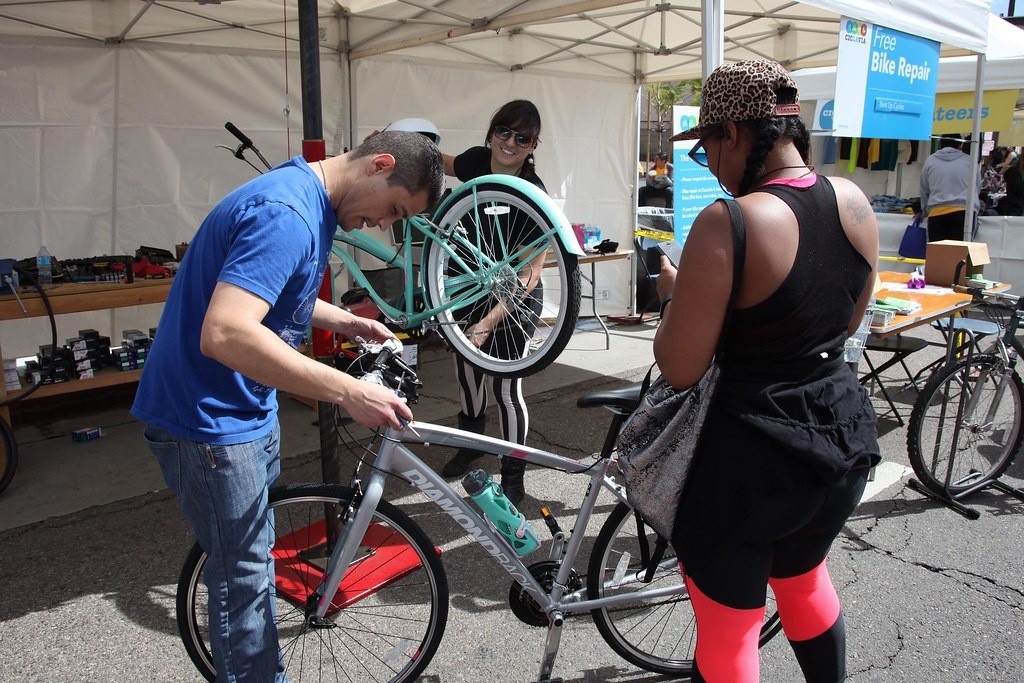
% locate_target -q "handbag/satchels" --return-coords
[898,213,927,259]
[619,364,721,541]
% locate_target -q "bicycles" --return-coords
[901,260,1024,500]
[176,340,781,683]
[214,118,588,378]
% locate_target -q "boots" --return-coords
[442,409,486,478]
[500,455,526,507]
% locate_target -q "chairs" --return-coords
[858,318,1003,404]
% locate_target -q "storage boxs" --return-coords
[924,238,990,288]
[2,325,157,391]
[72,427,107,442]
[389,188,458,264]
[335,329,422,379]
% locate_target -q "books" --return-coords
[875,296,922,325]
[965,275,1002,290]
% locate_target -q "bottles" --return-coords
[461,470,537,556]
[37,246,52,288]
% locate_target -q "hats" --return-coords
[667,59,801,142]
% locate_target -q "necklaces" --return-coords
[319,160,327,189]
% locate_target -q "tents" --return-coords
[776,11,1024,299]
[0,1,988,536]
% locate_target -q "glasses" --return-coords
[494,125,536,148]
[688,125,721,167]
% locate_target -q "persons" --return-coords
[128,128,444,683]
[360,99,549,511]
[655,59,881,683]
[986,145,1024,217]
[913,133,982,244]
[639,151,673,208]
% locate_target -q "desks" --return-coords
[874,211,1024,298]
[0,275,176,440]
[858,270,1012,427]
[542,249,635,350]
